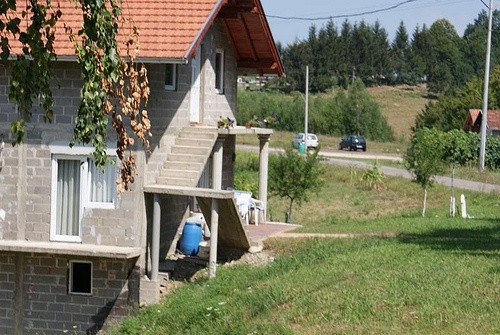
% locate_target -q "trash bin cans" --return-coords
[298,143,305,153]
[180,222,202,255]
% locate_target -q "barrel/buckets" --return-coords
[199,241,210,258]
[179,217,203,256]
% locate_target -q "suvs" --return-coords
[339,134,367,152]
[291,132,319,149]
